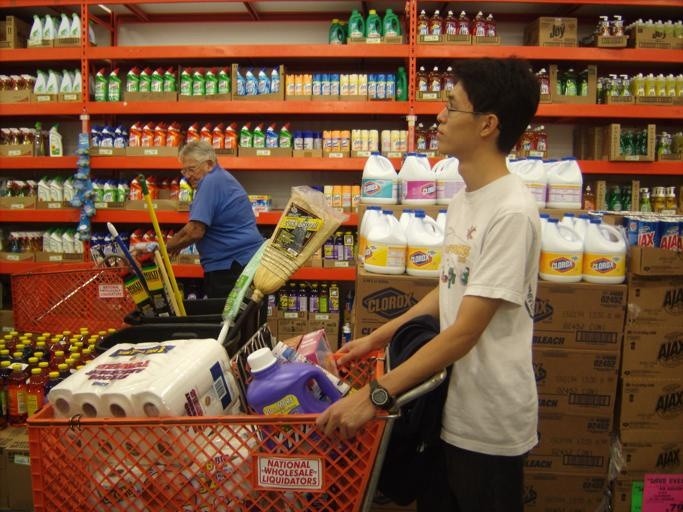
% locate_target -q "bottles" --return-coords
[634,73,683,99]
[657,131,682,160]
[618,126,648,156]
[416,124,439,152]
[293,129,408,152]
[267,283,342,315]
[507,123,550,161]
[5,228,41,251]
[582,186,594,210]
[314,230,357,262]
[625,20,682,50]
[417,66,455,94]
[414,10,498,40]
[534,65,590,100]
[0,327,118,426]
[285,73,396,99]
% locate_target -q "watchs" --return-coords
[366,378,399,415]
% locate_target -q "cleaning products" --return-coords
[84,3,414,286]
[1,0,84,271]
[414,2,680,288]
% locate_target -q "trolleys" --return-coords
[11,245,178,333]
[27,349,446,511]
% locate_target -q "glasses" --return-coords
[445,104,490,115]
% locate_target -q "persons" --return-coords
[159,139,266,304]
[314,56,543,510]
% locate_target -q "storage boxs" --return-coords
[0,422,33,512]
